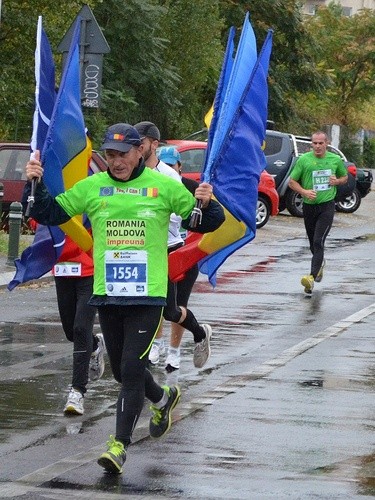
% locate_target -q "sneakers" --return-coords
[148,384,181,440]
[147,337,165,365]
[165,346,181,370]
[300,274,315,294]
[314,258,325,282]
[88,333,105,381]
[62,387,84,415]
[193,323,213,368]
[97,434,127,473]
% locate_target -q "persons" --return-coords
[132,121,213,369]
[147,146,198,370]
[22,124,226,474]
[26,125,105,416]
[288,131,347,295]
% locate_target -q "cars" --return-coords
[159,141,279,229]
[180,129,370,218]
[0,143,108,233]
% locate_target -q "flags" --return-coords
[7,28,92,288]
[167,11,273,288]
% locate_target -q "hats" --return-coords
[157,147,182,165]
[100,123,141,152]
[132,120,160,141]
